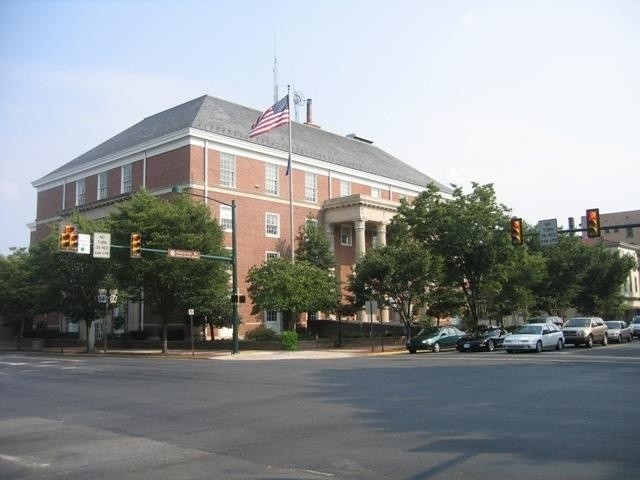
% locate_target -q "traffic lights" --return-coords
[231,293,239,303]
[238,294,246,303]
[131,232,142,259]
[585,208,602,238]
[511,218,523,246]
[59,222,79,253]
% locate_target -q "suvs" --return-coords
[559,316,609,349]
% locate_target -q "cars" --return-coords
[456,324,512,354]
[631,315,640,337]
[403,324,469,356]
[502,322,567,356]
[603,320,634,344]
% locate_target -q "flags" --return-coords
[249,94,290,138]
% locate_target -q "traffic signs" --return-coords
[93,231,112,260]
[537,218,560,246]
[78,233,92,255]
[167,249,202,260]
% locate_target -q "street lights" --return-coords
[171,184,244,356]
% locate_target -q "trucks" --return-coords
[526,316,564,330]
